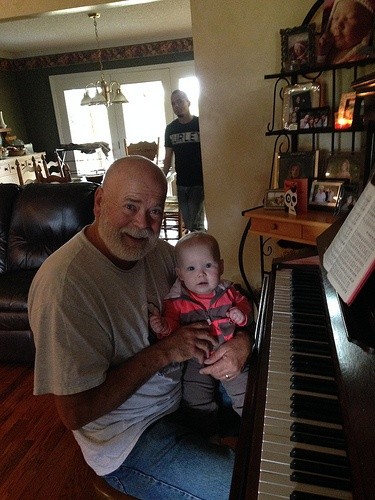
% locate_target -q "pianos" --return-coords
[228,171,375,500]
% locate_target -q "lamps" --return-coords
[81,12,129,107]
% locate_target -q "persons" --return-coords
[293,96,306,113]
[148,232,256,418]
[337,160,351,178]
[287,162,304,179]
[162,89,204,234]
[288,41,308,65]
[316,0,375,65]
[299,113,328,128]
[27,155,252,500]
[316,187,327,202]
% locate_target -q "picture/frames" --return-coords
[280,22,317,74]
[282,81,324,130]
[309,179,343,208]
[264,189,287,210]
[337,92,364,124]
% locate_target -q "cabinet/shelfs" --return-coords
[238,57,375,311]
[0,152,46,186]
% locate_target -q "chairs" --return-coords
[15,154,72,186]
[124,136,160,165]
[161,205,188,241]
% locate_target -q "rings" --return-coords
[225,373,230,379]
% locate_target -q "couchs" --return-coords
[0,183,99,354]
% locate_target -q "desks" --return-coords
[71,171,177,185]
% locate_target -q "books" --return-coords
[323,174,375,306]
